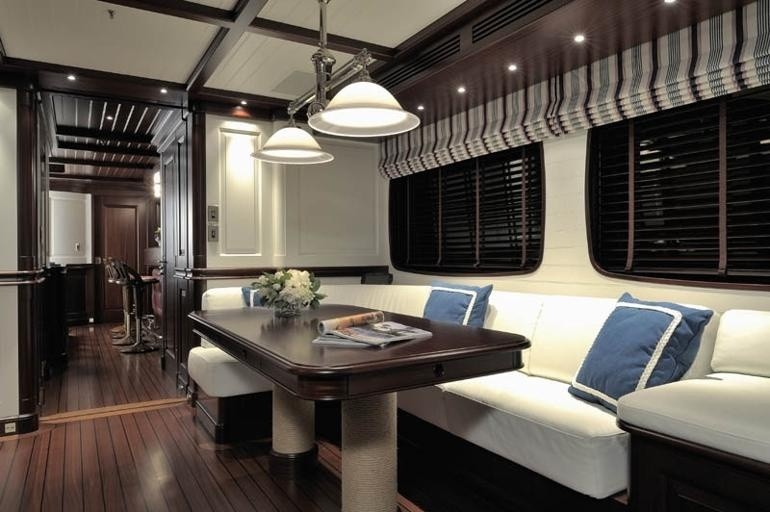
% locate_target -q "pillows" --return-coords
[422,279,493,329]
[567,291,715,416]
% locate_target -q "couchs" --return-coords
[186,284,721,511]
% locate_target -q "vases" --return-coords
[273,303,301,317]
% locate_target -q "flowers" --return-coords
[251,268,328,317]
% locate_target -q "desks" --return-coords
[189,301,532,512]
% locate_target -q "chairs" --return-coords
[102,256,160,354]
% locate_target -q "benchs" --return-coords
[613,306,770,511]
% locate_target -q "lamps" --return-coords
[250,0,423,166]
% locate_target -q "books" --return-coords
[311,309,433,351]
